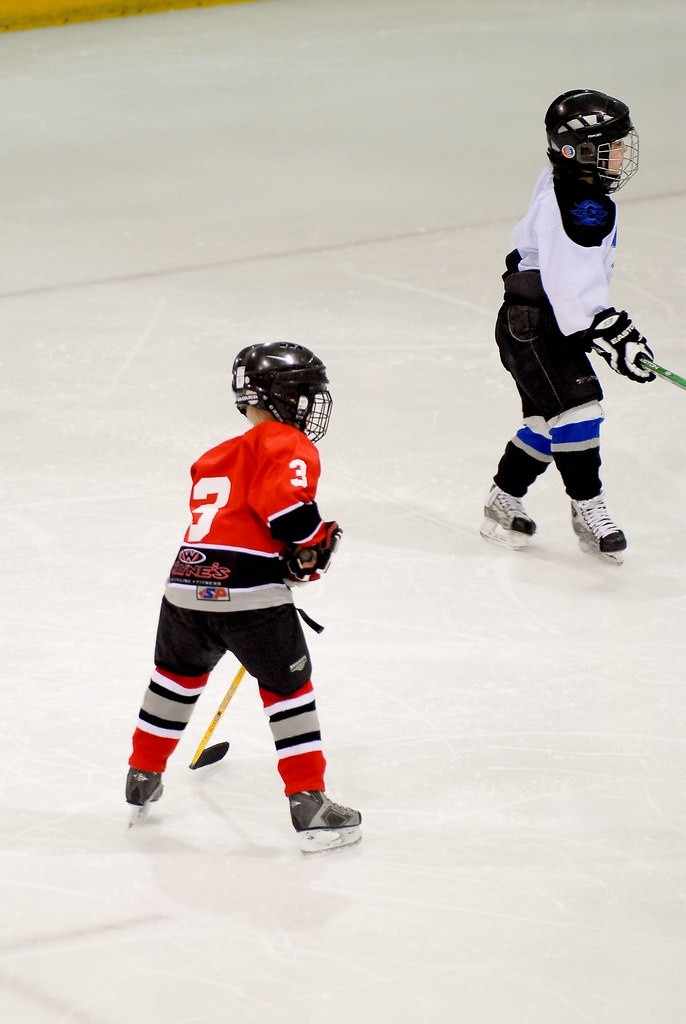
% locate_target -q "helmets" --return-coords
[545,89,631,193]
[232,340,329,432]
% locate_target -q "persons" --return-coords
[477,90,657,567]
[123,341,363,853]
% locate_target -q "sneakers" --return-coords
[571,491,627,565]
[126,769,164,828]
[289,790,364,854]
[479,485,536,551]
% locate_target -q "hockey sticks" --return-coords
[188,550,317,771]
[639,358,686,389]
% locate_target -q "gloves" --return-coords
[588,307,656,383]
